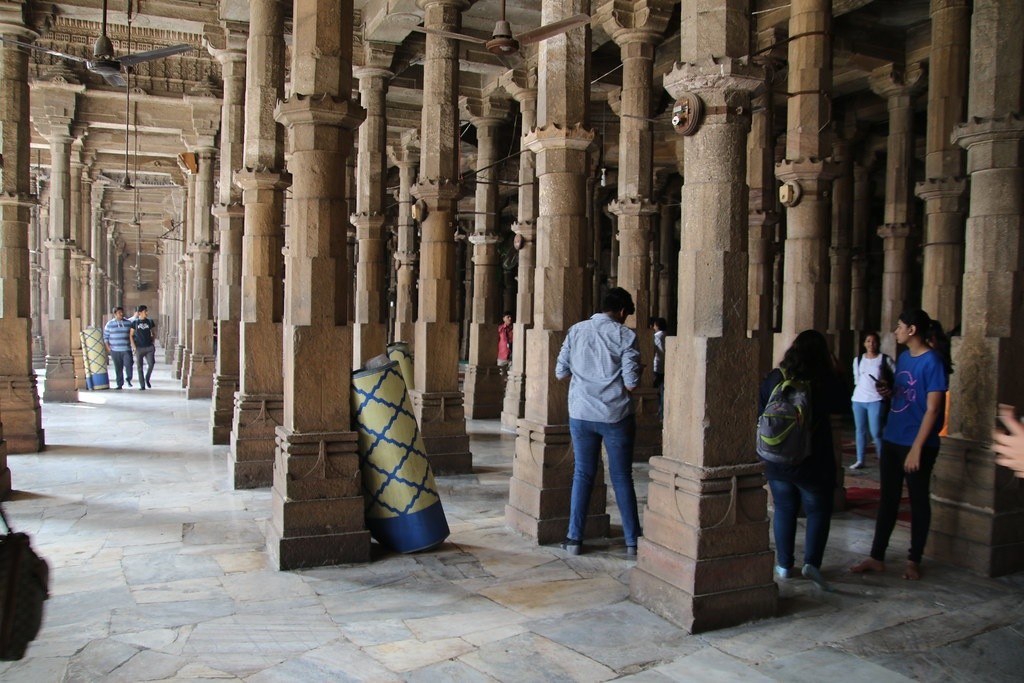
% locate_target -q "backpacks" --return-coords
[755,359,822,465]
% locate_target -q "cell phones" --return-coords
[868,374,886,389]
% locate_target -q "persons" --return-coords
[848,309,955,580]
[991,404,1024,482]
[555,287,642,555]
[497,310,512,387]
[758,329,844,592]
[127,306,139,321]
[130,304,156,389]
[103,307,134,390]
[653,318,670,421]
[849,333,896,469]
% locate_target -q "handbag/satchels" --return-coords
[1,510,50,661]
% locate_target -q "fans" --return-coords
[0,0,193,89]
[401,0,592,70]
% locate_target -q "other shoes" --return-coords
[560,540,582,556]
[627,545,637,555]
[776,565,792,578]
[802,564,828,588]
